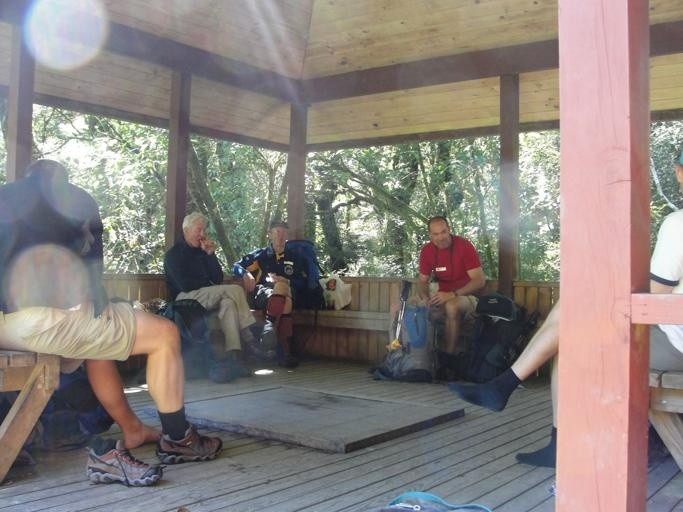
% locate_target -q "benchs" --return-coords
[0,309,683,484]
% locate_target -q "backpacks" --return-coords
[278,239,325,310]
[368,304,446,383]
[0,368,113,464]
[452,294,541,385]
[155,299,218,378]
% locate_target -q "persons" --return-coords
[161,210,275,380]
[0,159,223,464]
[231,218,308,368]
[366,215,484,382]
[445,144,683,467]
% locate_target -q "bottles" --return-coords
[427,269,440,300]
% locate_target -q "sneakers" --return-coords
[86,439,163,486]
[157,424,223,465]
